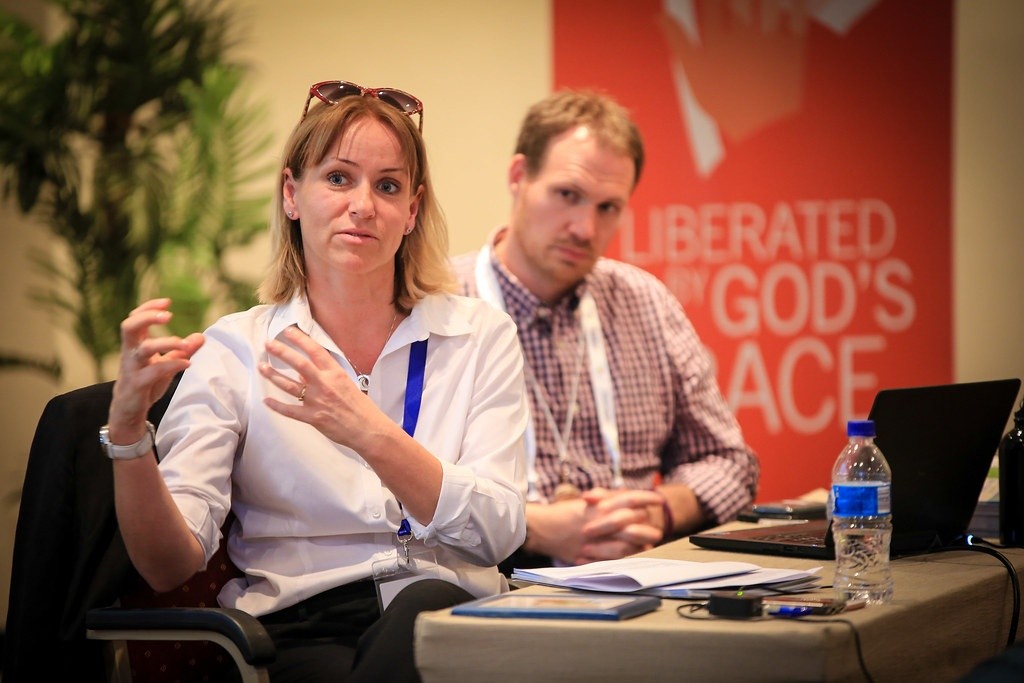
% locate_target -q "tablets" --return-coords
[450,593,663,623]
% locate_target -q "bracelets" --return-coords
[661,498,676,542]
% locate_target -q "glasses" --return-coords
[299,80,424,136]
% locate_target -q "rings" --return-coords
[298,385,309,401]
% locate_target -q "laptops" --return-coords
[688,378,1021,560]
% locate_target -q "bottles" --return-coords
[830,419,894,605]
[998,398,1024,548]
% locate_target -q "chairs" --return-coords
[46,367,274,683]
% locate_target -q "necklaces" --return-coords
[312,305,400,392]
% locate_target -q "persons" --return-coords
[427,88,763,591]
[107,79,530,683]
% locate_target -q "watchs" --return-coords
[99,420,156,460]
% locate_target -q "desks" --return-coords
[411,464,1024,683]
